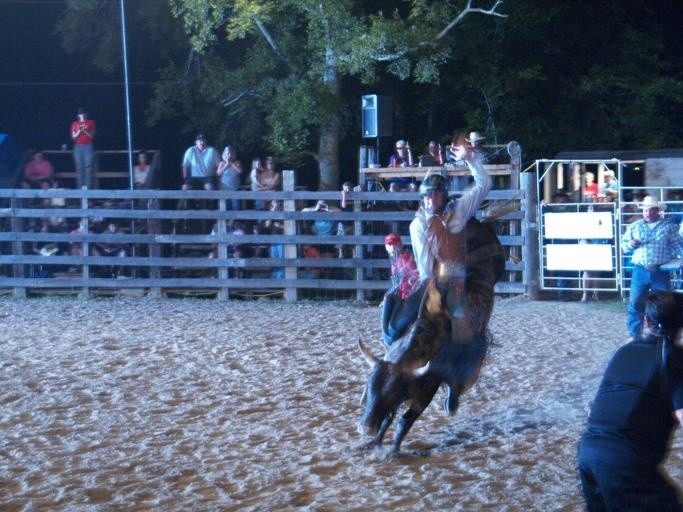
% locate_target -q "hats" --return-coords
[636,195,668,212]
[463,131,489,142]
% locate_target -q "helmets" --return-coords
[418,174,449,214]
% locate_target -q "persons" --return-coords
[409,131,495,420]
[579,289,682,512]
[383,234,421,304]
[618,194,683,344]
[412,138,448,198]
[549,168,620,302]
[381,139,417,211]
[337,179,409,211]
[442,130,492,192]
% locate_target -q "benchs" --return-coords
[16,172,151,298]
[170,183,307,300]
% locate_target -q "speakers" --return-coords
[361,94,392,138]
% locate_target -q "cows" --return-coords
[356,199,522,458]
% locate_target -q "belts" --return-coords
[633,263,663,272]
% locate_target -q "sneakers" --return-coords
[446,383,461,417]
